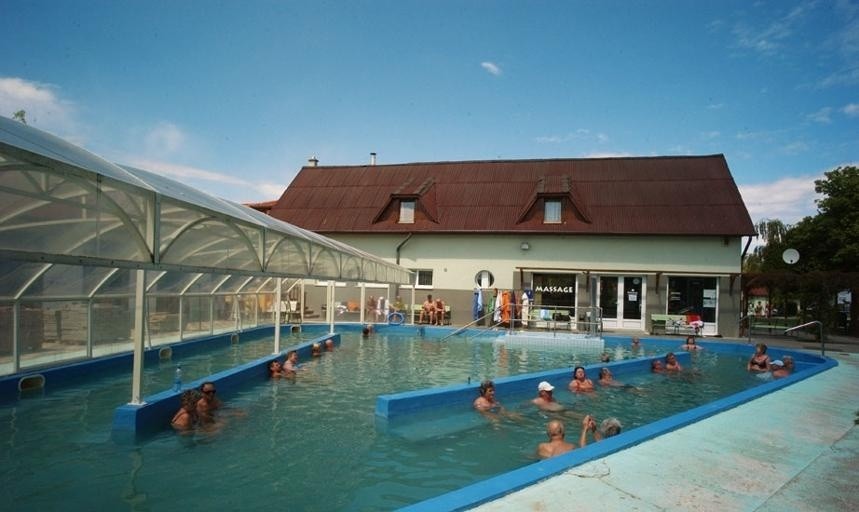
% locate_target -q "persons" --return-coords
[166,388,222,443]
[310,342,321,356]
[567,367,608,405]
[518,420,578,463]
[321,339,343,354]
[267,359,297,380]
[472,380,534,433]
[281,351,313,376]
[740,298,775,319]
[597,334,802,400]
[578,412,622,447]
[195,381,249,431]
[324,287,447,338]
[529,381,586,427]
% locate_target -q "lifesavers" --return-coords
[387,313,404,325]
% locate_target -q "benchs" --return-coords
[528,309,570,330]
[324,297,387,322]
[650,313,702,336]
[390,304,451,327]
[749,315,798,338]
[267,300,301,324]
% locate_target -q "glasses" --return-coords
[202,390,216,394]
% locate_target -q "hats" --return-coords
[769,360,784,366]
[538,381,555,392]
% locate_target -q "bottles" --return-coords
[173,364,183,392]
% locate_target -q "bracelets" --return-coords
[592,425,597,432]
